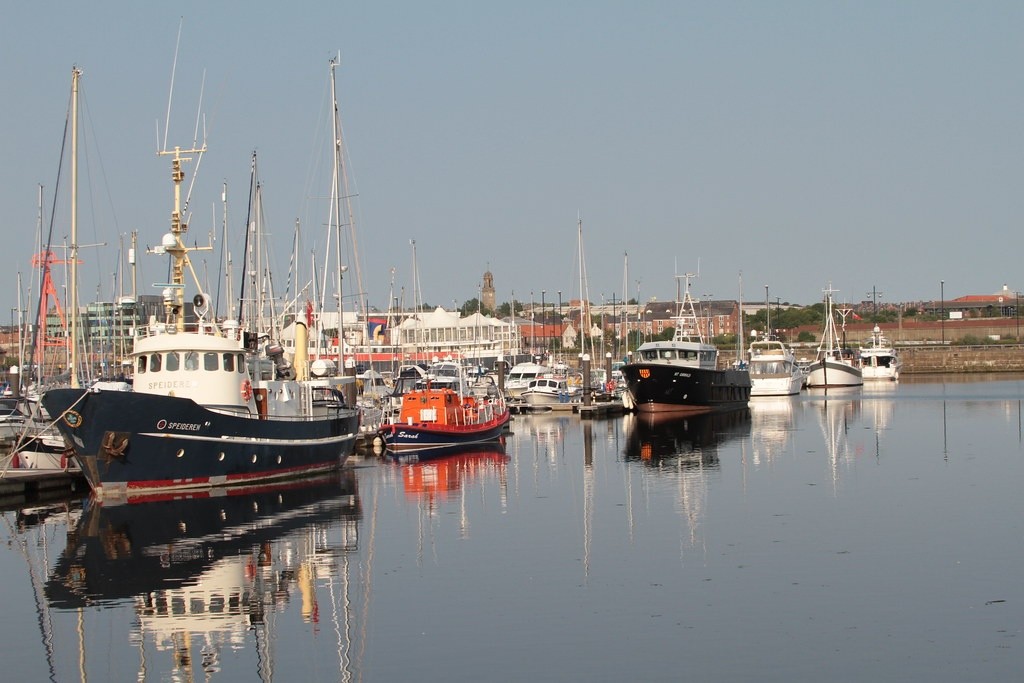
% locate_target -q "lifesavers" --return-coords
[240,380,253,401]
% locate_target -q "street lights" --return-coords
[541,290,546,354]
[394,297,399,358]
[1012,291,1022,343]
[557,291,563,359]
[765,285,769,337]
[940,280,946,347]
[867,294,883,338]
[775,296,782,342]
[11,307,17,371]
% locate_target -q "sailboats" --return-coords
[41,26,363,509]
[619,256,752,422]
[0,69,900,479]
[378,239,511,456]
